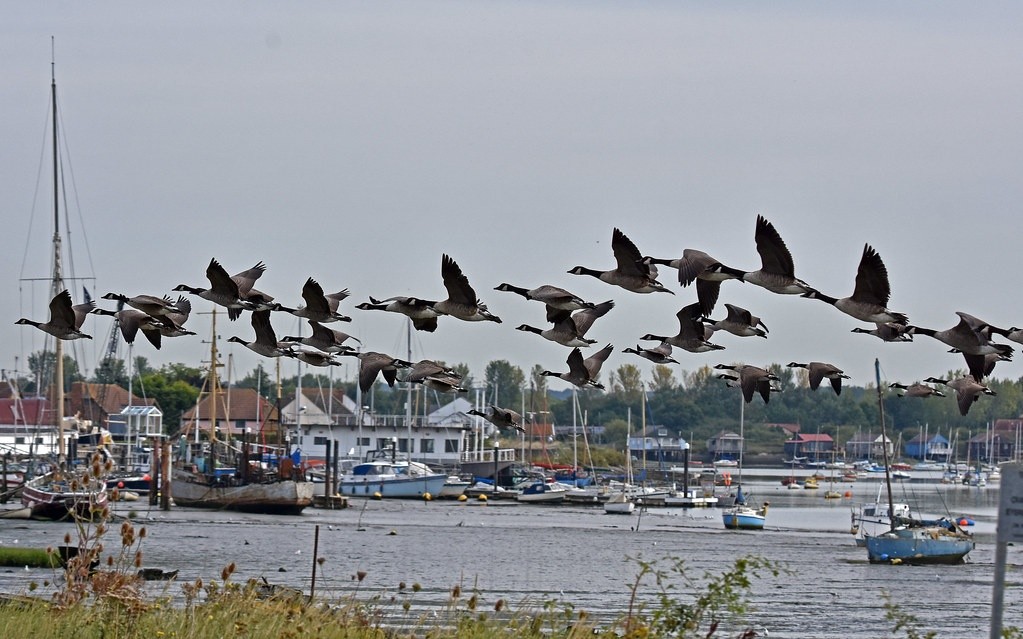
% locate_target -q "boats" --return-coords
[306,471,341,496]
[893,470,910,478]
[842,476,856,482]
[0,452,27,495]
[864,463,889,472]
[604,489,634,513]
[855,480,912,546]
[107,470,152,492]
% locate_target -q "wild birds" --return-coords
[621,300,769,364]
[227,310,362,367]
[272,277,353,324]
[492,282,615,347]
[334,351,467,394]
[923,375,998,416]
[354,252,502,333]
[90,292,196,350]
[566,227,676,296]
[889,383,946,398]
[12,289,95,341]
[714,363,783,405]
[703,213,810,295]
[901,311,1023,384]
[786,362,851,396]
[800,242,913,343]
[635,248,745,317]
[172,256,275,321]
[466,404,530,434]
[540,342,614,391]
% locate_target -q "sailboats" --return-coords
[519,384,608,502]
[825,451,841,498]
[804,423,823,489]
[18,37,108,511]
[865,360,976,562]
[609,381,735,505]
[890,421,945,472]
[782,421,799,489]
[280,385,519,496]
[337,315,449,496]
[722,393,765,528]
[941,425,985,486]
[165,310,317,506]
